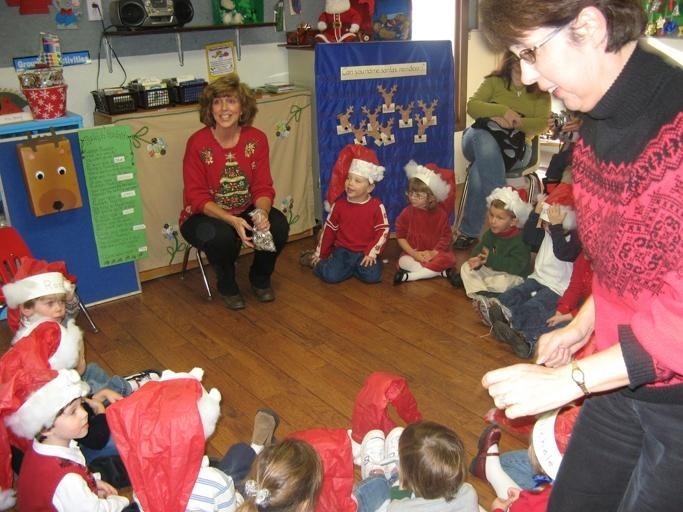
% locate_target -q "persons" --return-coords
[479,0,683,511]
[445,186,534,301]
[314,0,361,44]
[454,49,551,251]
[298,142,387,284]
[178,72,290,309]
[473,182,582,358]
[0,252,596,510]
[394,159,459,283]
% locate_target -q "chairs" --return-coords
[453,135,541,233]
[0,226,99,337]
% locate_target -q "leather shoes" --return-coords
[469,424,502,477]
[393,266,411,284]
[441,266,462,288]
[218,290,245,309]
[251,281,275,303]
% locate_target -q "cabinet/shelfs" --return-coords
[286,40,454,227]
[93,86,314,284]
[0,112,83,138]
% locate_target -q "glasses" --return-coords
[404,189,426,200]
[507,26,564,61]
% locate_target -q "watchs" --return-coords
[571,363,592,402]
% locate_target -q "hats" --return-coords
[348,370,423,466]
[323,144,385,215]
[1,258,91,441]
[486,185,534,228]
[105,365,221,512]
[532,402,582,480]
[404,158,456,218]
[539,180,577,230]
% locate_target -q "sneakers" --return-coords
[251,408,278,447]
[453,233,476,250]
[360,426,406,483]
[299,248,315,266]
[123,370,161,390]
[473,294,493,328]
[492,321,530,358]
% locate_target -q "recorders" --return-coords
[110,0,195,29]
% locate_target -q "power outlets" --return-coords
[86,1,104,20]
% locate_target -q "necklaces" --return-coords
[511,81,527,98]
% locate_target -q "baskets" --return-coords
[129,86,175,109]
[177,82,208,105]
[90,87,138,115]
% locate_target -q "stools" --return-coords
[177,234,215,300]
